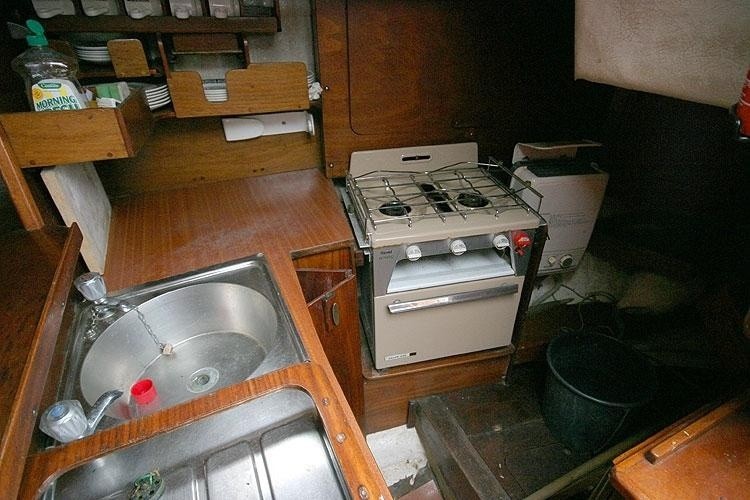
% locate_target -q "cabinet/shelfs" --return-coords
[1,1,315,170]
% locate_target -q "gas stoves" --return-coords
[348,157,541,248]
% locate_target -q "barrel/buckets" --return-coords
[545,329,659,455]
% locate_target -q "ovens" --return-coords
[373,230,537,367]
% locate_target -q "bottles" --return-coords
[11,21,89,111]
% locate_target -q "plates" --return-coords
[202,60,320,102]
[124,82,171,114]
[76,38,124,62]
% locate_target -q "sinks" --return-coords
[34,253,310,452]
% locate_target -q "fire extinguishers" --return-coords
[736,70,750,137]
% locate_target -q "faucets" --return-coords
[38,387,124,443]
[72,271,133,317]
